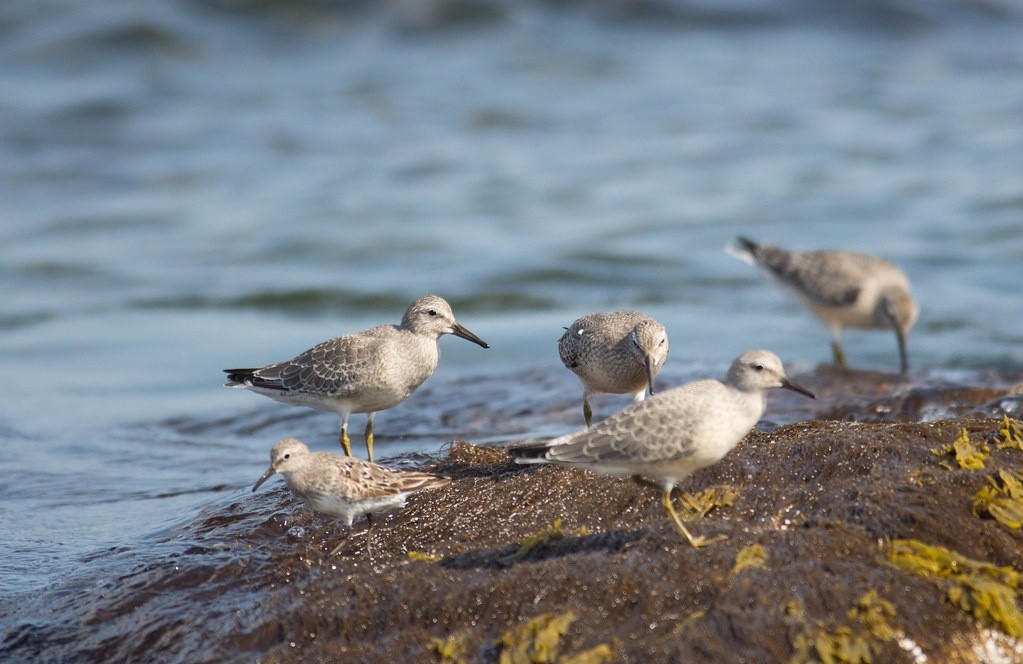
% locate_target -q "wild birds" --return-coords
[730,235,919,382]
[506,347,817,551]
[222,294,490,466]
[556,309,669,428]
[252,437,453,563]
